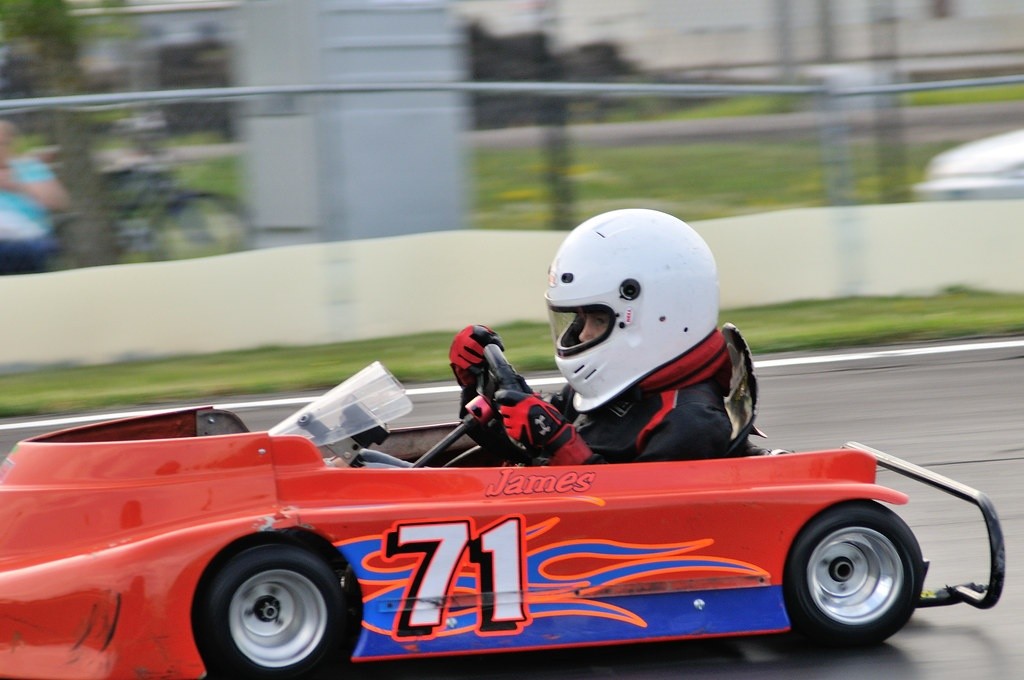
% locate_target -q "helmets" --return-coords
[546,209,717,411]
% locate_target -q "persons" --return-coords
[323,208,732,468]
[0,121,69,275]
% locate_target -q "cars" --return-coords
[906,128,1024,205]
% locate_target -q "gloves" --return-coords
[450,326,506,387]
[495,388,592,466]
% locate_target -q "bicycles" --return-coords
[94,107,256,259]
